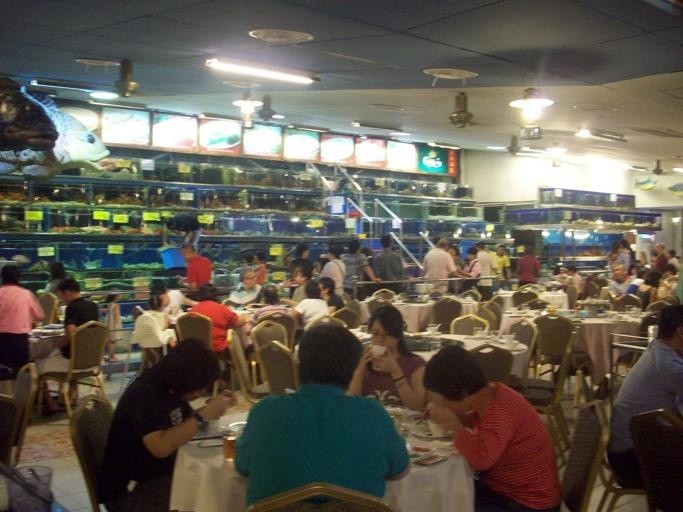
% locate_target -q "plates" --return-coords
[407,453,423,462]
[411,427,456,441]
[199,120,241,150]
[321,137,352,162]
[504,311,529,317]
[226,422,247,430]
[286,133,319,157]
[408,445,436,454]
[57,106,98,132]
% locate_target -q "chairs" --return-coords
[1,264,683,511]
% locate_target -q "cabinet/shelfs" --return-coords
[0,136,681,265]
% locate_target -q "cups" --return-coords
[425,323,501,342]
[222,430,237,463]
[521,303,529,312]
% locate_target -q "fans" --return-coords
[441,88,505,130]
[251,91,311,124]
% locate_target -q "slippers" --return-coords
[54,396,75,406]
[42,406,67,420]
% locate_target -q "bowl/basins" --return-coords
[425,418,450,437]
[501,334,516,342]
[507,306,521,315]
[367,344,387,359]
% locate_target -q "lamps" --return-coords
[205,53,321,88]
[232,85,264,120]
[508,87,559,125]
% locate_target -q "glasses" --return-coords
[368,330,393,342]
